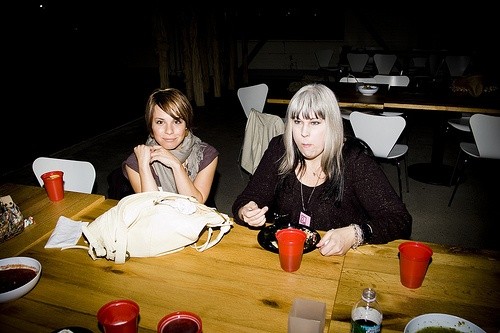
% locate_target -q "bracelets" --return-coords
[350,224,363,250]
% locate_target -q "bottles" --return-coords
[350,287,382,333]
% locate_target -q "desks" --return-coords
[254,81,388,109]
[384,86,500,118]
[0,184,500,333]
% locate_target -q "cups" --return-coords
[288,297,326,333]
[276,229,306,273]
[97,300,139,333]
[397,241,433,289]
[40,170,65,202]
[157,310,204,333]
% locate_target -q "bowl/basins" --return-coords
[0,257,42,302]
[404,313,487,333]
[358,85,378,96]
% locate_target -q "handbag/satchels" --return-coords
[82,191,233,264]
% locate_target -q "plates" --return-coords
[51,327,93,333]
[256,222,322,255]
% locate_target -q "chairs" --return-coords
[32,157,96,194]
[237,53,500,207]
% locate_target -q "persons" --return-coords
[122,88,219,203]
[232,84,413,257]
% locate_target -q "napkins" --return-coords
[44,216,89,248]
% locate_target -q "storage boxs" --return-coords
[288,297,326,333]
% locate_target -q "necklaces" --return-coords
[308,164,322,176]
